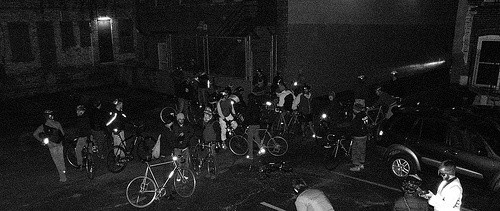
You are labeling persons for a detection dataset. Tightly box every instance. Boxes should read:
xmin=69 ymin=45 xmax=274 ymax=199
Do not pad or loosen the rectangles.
xmin=390 ymin=174 xmax=429 ymax=211
xmin=291 ymin=178 xmax=335 ymax=211
xmin=33 ymin=66 xmax=411 ymax=182
xmin=419 ymin=160 xmax=463 ymax=211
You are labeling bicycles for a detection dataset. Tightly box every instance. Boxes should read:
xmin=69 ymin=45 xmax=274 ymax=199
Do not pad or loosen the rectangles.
xmin=106 ymin=129 xmax=158 ymax=174
xmin=126 ymin=155 xmax=196 ymax=209
xmin=66 ymin=130 xmax=96 ymax=180
xmin=325 ymin=135 xmax=354 ymax=171
xmin=272 ymin=110 xmax=301 ymax=138
xmin=159 ymin=99 xmax=202 ymax=126
xmin=228 ymin=126 xmax=289 ymax=157
xmin=189 ymin=136 xmax=217 ymax=179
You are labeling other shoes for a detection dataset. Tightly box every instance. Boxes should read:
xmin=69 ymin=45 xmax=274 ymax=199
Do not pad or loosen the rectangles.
xmin=258 ymin=147 xmax=265 ymax=154
xmin=222 ymin=144 xmax=227 ymax=150
xmin=350 ymin=166 xmax=360 ymax=171
xmin=359 ymin=164 xmax=364 ymax=169
xmin=246 ymin=156 xmax=253 ymax=160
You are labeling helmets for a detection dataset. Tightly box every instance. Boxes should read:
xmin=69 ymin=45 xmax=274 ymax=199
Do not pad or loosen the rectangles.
xmin=439 ymin=161 xmax=455 ymax=175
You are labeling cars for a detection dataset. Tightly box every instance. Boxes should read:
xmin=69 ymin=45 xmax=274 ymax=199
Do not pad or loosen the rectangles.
xmin=370 ymin=114 xmax=500 ymax=198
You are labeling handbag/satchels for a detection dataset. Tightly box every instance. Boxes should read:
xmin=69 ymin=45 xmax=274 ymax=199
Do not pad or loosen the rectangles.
xmin=175 ymin=148 xmax=190 ymax=169
xmin=152 ymin=134 xmax=162 ymax=159
xmin=43 ymin=123 xmax=63 ymax=144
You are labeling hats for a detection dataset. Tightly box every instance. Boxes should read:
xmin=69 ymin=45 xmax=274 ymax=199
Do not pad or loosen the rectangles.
xmin=328 ymin=91 xmax=335 ymax=97
xmin=177 ymin=113 xmax=185 ymax=121
xmin=43 ymin=110 xmax=54 ymax=114
xmin=277 ymin=79 xmax=284 ymax=84
xmin=204 ymin=107 xmax=213 ymax=115
xmin=353 ymin=104 xmax=363 ymax=111
xmin=374 ymin=87 xmax=384 ymax=91
xmin=76 ymin=105 xmax=86 ymax=110
xmin=304 ymin=87 xmax=309 ymax=90
